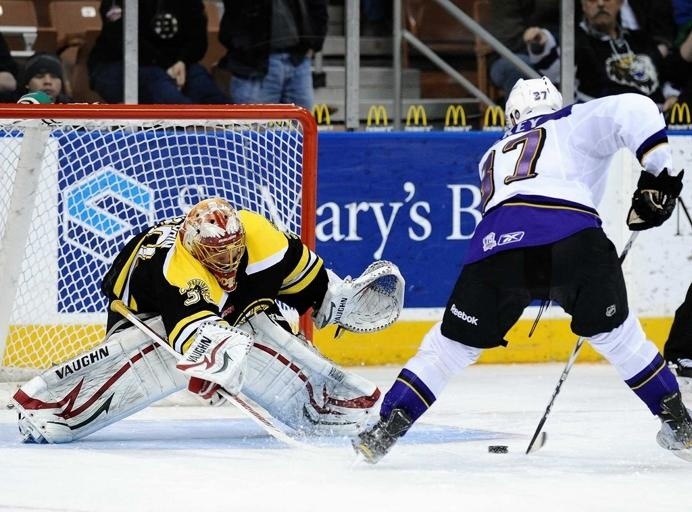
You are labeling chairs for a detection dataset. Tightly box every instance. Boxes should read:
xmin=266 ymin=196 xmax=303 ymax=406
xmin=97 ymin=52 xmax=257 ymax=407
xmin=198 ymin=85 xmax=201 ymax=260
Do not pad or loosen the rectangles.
xmin=0 ymin=0 xmax=241 ymax=113
xmin=392 ymin=1 xmax=540 ymax=131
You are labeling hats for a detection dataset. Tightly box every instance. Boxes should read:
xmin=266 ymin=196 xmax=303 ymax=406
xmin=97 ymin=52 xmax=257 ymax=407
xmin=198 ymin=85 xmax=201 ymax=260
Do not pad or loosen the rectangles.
xmin=21 ymin=51 xmax=64 ymax=86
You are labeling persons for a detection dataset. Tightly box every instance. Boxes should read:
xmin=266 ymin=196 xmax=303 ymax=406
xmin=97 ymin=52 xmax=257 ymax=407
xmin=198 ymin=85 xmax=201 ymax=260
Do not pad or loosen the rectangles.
xmin=665 ymin=280 xmax=690 ymax=378
xmin=342 ymin=1 xmax=413 ymax=69
xmin=215 ymin=1 xmax=329 ymax=112
xmin=349 ymin=74 xmax=691 ymax=462
xmin=1 ymin=32 xmax=74 ymax=104
xmin=483 ymin=0 xmax=692 ymax=125
xmin=11 ymin=199 xmax=405 ymax=444
xmin=88 ymin=1 xmax=235 ymax=104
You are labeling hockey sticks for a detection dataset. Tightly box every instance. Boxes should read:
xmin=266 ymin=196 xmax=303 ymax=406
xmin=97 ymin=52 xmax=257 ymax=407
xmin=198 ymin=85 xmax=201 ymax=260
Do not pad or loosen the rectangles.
xmin=111 ymin=301 xmax=360 ymax=449
xmin=527 ymin=227 xmax=639 ymax=454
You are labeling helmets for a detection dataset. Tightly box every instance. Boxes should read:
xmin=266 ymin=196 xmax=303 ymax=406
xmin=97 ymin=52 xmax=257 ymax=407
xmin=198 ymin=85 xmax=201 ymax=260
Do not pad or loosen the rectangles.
xmin=177 ymin=195 xmax=250 ymax=294
xmin=502 ymin=75 xmax=567 ymax=131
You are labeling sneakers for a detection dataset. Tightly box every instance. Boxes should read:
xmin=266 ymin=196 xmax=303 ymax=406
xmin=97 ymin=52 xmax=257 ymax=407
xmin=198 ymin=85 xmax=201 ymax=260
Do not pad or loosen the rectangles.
xmin=655 ymin=390 xmax=692 ymax=449
xmin=671 ymin=358 xmax=692 ymax=378
xmin=350 ymin=407 xmax=414 ymax=465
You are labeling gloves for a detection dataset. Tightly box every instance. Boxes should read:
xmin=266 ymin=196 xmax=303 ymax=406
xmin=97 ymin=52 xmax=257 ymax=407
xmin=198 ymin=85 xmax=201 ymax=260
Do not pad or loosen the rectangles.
xmin=626 ymin=168 xmax=686 ymax=232
